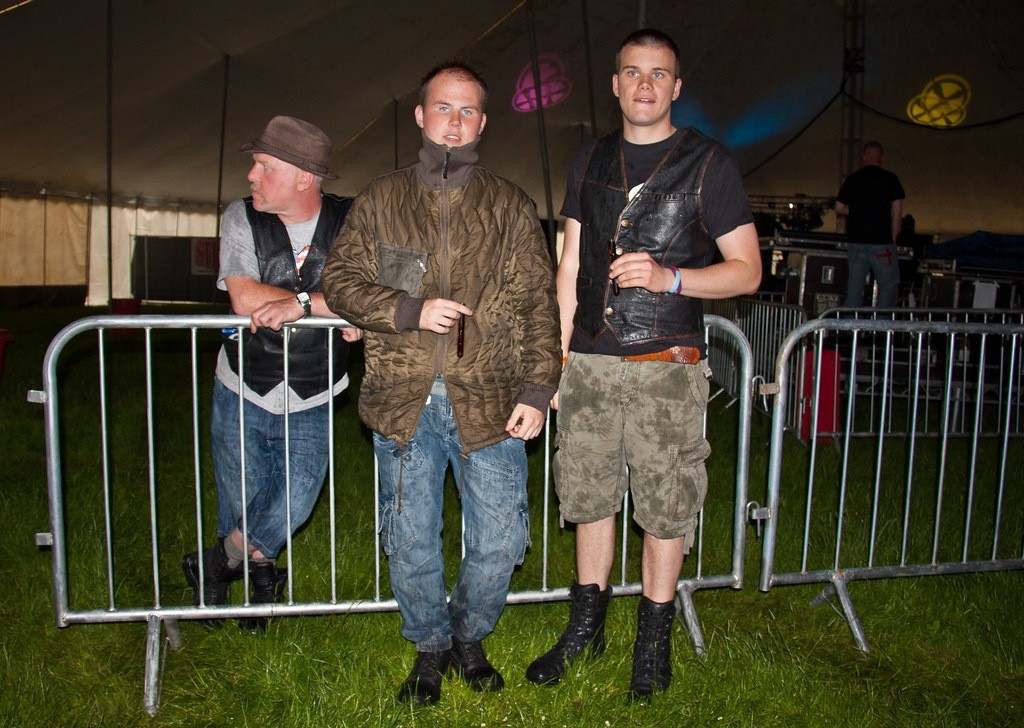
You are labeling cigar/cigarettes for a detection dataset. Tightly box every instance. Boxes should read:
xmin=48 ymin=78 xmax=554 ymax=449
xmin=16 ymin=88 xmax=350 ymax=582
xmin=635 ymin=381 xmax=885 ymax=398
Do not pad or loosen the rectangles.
xmin=457 ymin=303 xmax=466 ymax=358
xmin=610 ymin=239 xmax=620 ymax=296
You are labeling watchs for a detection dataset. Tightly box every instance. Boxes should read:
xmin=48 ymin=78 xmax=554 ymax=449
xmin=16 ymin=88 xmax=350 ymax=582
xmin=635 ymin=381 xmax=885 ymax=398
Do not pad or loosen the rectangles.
xmin=296 ymin=291 xmax=312 ymax=316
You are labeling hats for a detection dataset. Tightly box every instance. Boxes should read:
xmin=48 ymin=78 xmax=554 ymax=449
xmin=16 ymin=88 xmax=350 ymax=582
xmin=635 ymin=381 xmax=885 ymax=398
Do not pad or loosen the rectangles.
xmin=238 ymin=115 xmax=339 ymax=180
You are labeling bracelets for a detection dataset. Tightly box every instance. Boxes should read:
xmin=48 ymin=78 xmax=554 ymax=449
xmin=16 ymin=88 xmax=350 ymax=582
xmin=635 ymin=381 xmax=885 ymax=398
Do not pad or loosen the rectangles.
xmin=667 ymin=267 xmax=681 ymax=296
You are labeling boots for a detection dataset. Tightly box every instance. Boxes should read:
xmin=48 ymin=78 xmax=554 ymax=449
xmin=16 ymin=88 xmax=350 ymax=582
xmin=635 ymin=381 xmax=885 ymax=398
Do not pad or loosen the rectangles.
xmin=526 ymin=580 xmax=613 ymax=687
xmin=626 ymin=597 xmax=677 ymax=705
xmin=182 ymin=536 xmax=244 ymax=631
xmin=238 ymin=561 xmax=288 ymax=633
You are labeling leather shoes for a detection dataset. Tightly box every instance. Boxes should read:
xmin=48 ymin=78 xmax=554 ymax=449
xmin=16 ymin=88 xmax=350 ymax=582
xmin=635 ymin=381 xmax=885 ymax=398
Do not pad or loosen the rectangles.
xmin=451 ymin=635 xmax=505 ymax=693
xmin=398 ymin=651 xmax=450 ymax=707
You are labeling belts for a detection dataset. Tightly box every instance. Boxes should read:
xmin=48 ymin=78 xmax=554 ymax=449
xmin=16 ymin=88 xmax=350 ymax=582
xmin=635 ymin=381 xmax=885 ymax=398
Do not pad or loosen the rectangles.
xmin=623 ymin=346 xmax=700 ymax=366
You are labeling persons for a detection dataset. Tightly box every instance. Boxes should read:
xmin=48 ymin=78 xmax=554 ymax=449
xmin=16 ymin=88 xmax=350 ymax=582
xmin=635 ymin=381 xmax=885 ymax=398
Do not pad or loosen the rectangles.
xmin=321 ymin=65 xmax=563 ymax=703
xmin=525 ymin=31 xmax=762 ymax=704
xmin=183 ymin=113 xmax=363 ymax=634
xmin=834 ymin=141 xmax=906 ymax=320
